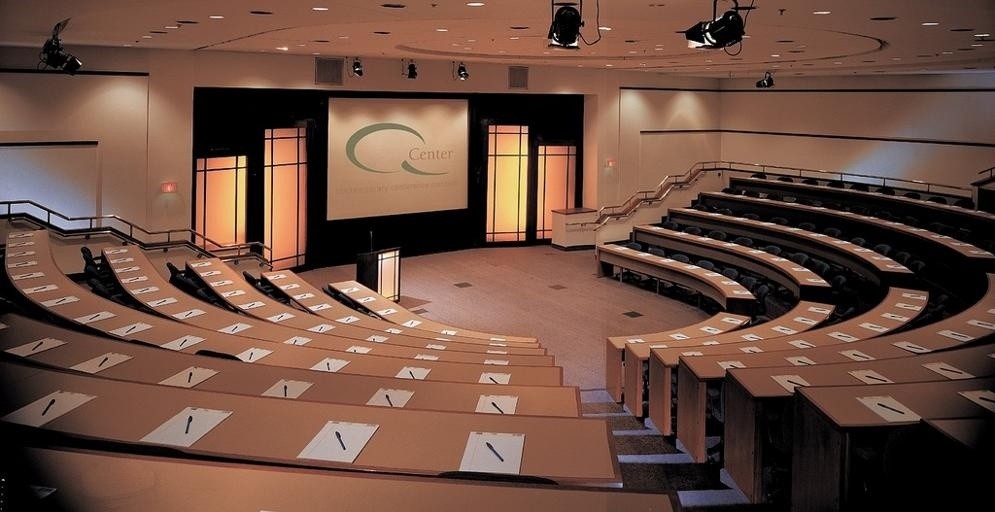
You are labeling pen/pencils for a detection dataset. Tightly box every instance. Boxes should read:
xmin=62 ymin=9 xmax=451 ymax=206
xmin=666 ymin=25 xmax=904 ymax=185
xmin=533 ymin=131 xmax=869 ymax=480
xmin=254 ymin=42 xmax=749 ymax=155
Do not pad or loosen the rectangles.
xmin=910 ymin=295 xmax=921 ymax=297
xmin=229 ymin=292 xmax=236 ymax=295
xmin=489 ymin=377 xmax=498 ymax=384
xmin=977 ymin=323 xmax=992 ymax=326
xmin=951 ymin=333 xmax=968 ymax=338
xmin=801 ymin=319 xmax=811 ymax=321
xmin=877 ymin=402 xmax=904 ymax=414
xmin=729 ymin=364 xmax=738 ymax=368
xmin=185 ymin=415 xmax=192 ymax=433
xmin=870 ymin=326 xmax=881 ymax=328
xmin=409 ymin=371 xmax=415 ymax=379
xmin=787 ymin=380 xmax=805 ymax=387
xmin=42 ymin=399 xmax=55 ymax=415
xmin=32 ymin=341 xmax=43 ymax=351
xmin=34 ymin=287 xmax=46 ymax=291
xmin=16 ymin=261 xmax=28 ymax=266
xmin=491 ymin=402 xmax=503 ymax=414
xmin=21 ymin=274 xmax=33 ymax=277
xmin=56 ymin=298 xmax=65 ymax=303
xmin=199 ymin=263 xmax=205 ymax=265
xmin=319 ymin=326 xmax=323 ymax=331
xmin=140 ymin=288 xmax=149 ymax=292
xmin=207 ymin=272 xmax=213 ymax=275
xmin=797 ymin=360 xmax=811 ymax=366
xmin=16 ymin=243 xmax=25 ymax=245
xmin=248 ymin=304 xmax=254 ymax=307
xmin=15 ymin=252 xmax=25 ymax=256
xmin=283 ymin=384 xmax=287 ymax=396
xmin=278 ymin=315 xmax=283 ymax=320
xmin=119 ymin=258 xmax=126 ymax=261
xmin=232 ymin=326 xmax=238 ymax=331
xmin=385 ymin=394 xmax=393 ymax=407
xmin=800 ymin=343 xmax=813 ymax=348
xmin=815 ymin=309 xmax=826 ymax=311
xmin=939 ymin=368 xmax=962 ymax=374
xmin=891 ymin=315 xmax=903 ymax=318
xmin=185 ymin=312 xmax=192 ymax=317
xmin=979 ymin=397 xmax=995 ymax=403
xmin=124 ymin=268 xmax=131 ymax=270
xmin=126 ymin=326 xmax=136 ymax=333
xmin=749 ymin=350 xmax=756 ymax=352
xmin=293 ymin=340 xmax=296 ymax=345
xmin=865 ymin=376 xmax=887 ymax=382
xmin=157 ymin=300 xmax=166 ymax=304
xmin=750 ymin=336 xmax=762 ymax=340
xmin=112 ymin=250 xmax=119 ymax=253
xmin=188 ymin=372 xmax=192 ymax=383
xmin=906 ymin=346 xmax=923 ymax=351
xmin=837 ymin=334 xmax=851 ymax=338
xmin=335 ymin=431 xmax=346 ymax=451
xmin=852 ymin=354 xmax=869 ymax=359
xmin=904 ymin=305 xmax=915 ymax=307
xmin=485 ymin=442 xmax=504 ymax=462
xmin=327 ymin=362 xmax=330 ymax=370
xmin=129 ymin=278 xmax=139 ymax=281
xmin=89 ymin=314 xmax=100 ymax=320
xmin=780 ymin=328 xmax=791 ymax=331
xmin=218 ymin=281 xmax=224 ymax=285
xmin=17 ymin=234 xmax=24 ymax=236
xmin=180 ymin=339 xmax=186 ymax=346
xmin=249 ymin=352 xmax=253 ymax=360
xmin=98 ymin=357 xmax=108 ymax=367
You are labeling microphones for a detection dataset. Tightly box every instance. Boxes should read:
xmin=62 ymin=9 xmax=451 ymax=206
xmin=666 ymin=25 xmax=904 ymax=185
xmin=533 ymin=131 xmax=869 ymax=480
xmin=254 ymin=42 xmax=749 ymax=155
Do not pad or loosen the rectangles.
xmin=370 ymin=231 xmax=372 ymax=253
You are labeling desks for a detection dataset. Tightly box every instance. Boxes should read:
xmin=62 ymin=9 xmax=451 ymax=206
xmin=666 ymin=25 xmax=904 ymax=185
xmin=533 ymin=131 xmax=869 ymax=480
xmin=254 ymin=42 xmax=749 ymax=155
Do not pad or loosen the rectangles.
xmin=550 ymin=206 xmax=599 ymax=252
xmin=0 ymin=171 xmax=995 ymax=510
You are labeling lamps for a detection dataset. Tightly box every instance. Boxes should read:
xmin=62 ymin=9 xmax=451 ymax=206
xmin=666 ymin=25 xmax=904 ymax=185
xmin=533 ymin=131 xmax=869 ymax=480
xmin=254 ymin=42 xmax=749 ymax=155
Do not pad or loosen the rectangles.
xmin=344 ymin=55 xmax=363 ymax=78
xmin=35 ymin=23 xmax=83 ymax=76
xmin=161 ymin=182 xmax=179 ymax=193
xmin=451 ymin=59 xmax=469 ymax=82
xmin=676 ymin=1 xmax=755 ymax=55
xmin=546 ymin=0 xmax=602 ymax=52
xmin=400 ymin=59 xmax=419 ymax=79
xmin=755 ymin=71 xmax=774 ymax=88
